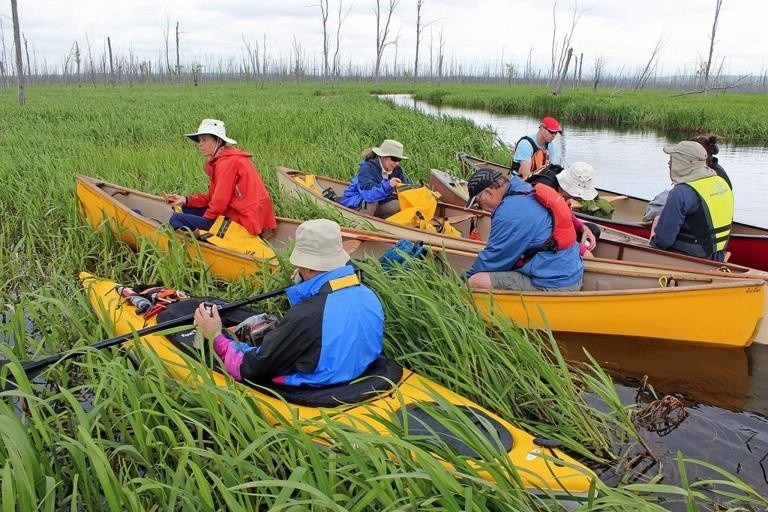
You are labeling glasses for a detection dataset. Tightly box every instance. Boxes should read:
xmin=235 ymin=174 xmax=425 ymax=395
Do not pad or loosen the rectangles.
xmin=476 ymin=193 xmax=483 ymax=209
xmin=389 ymin=156 xmax=402 ymax=162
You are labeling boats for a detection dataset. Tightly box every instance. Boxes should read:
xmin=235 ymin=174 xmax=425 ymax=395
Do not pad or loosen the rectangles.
xmin=275 ymin=166 xmax=768 ymax=347
xmin=427 ymin=167 xmax=653 ymax=249
xmin=79 ymin=271 xmax=598 ymax=512
xmin=456 ymin=150 xmax=768 ymax=273
xmin=743 ymin=342 xmax=768 ymax=422
xmin=73 ymin=172 xmax=766 ymax=349
xmin=485 ymin=330 xmax=749 ymax=413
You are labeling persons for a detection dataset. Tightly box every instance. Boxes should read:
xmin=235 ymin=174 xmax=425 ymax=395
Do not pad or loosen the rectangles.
xmin=193 ymin=218 xmax=384 ymax=389
xmin=688 ymin=134 xmax=733 ymax=191
xmin=648 ymin=141 xmax=733 ymax=262
xmin=522 ymin=163 xmax=601 ymax=260
xmin=507 ymin=117 xmax=562 ymax=182
xmin=452 ymin=168 xmax=584 ymax=294
xmin=339 ymin=139 xmax=409 ymax=219
xmin=165 ymin=119 xmax=276 ymax=244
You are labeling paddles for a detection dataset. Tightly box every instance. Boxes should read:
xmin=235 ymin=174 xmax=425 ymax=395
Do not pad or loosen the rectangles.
xmin=0 ymin=260 xmax=373 ymax=398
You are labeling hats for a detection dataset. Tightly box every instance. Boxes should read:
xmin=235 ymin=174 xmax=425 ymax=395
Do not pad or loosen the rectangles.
xmin=372 ymin=140 xmax=408 ymax=160
xmin=663 ymin=142 xmax=708 ymax=160
xmin=290 ymin=219 xmax=350 ymax=272
xmin=464 ymin=167 xmax=504 ymax=210
xmin=556 ymin=162 xmax=599 ymax=201
xmin=185 ymin=118 xmax=238 ymax=144
xmin=541 ymin=117 xmax=563 ymax=134
